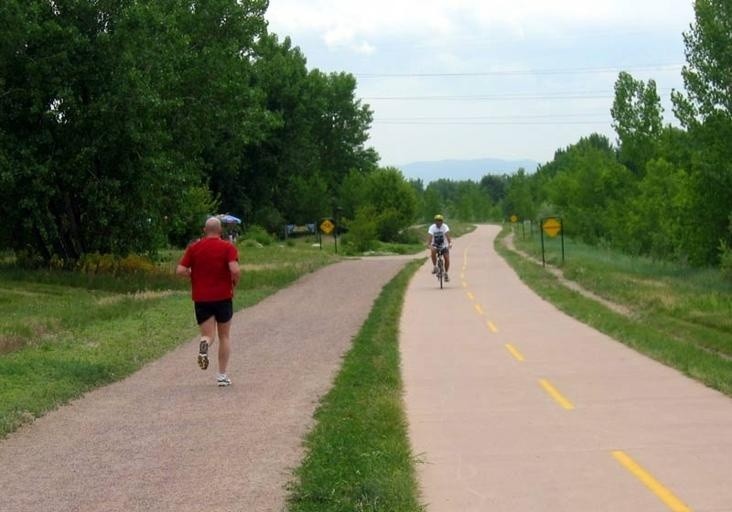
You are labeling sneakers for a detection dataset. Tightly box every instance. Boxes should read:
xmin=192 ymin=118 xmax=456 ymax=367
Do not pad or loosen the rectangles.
xmin=431 ymin=266 xmax=438 ymax=274
xmin=197 ymin=337 xmax=211 ymax=370
xmin=444 ymin=273 xmax=450 ymax=282
xmin=216 ymin=376 xmax=233 ymax=387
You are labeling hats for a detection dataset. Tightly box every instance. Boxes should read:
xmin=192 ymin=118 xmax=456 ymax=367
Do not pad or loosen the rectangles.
xmin=434 ymin=214 xmax=444 ymax=221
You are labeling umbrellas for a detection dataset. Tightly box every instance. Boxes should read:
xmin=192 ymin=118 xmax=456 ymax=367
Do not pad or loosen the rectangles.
xmin=208 ymin=212 xmax=243 ymax=239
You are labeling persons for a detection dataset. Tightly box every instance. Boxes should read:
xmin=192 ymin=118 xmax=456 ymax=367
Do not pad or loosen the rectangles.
xmin=426 ymin=214 xmax=453 ymax=283
xmin=176 ymin=216 xmax=241 ymax=387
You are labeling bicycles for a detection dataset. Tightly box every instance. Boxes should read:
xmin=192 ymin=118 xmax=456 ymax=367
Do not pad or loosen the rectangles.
xmin=426 ymin=245 xmax=453 ymax=288
